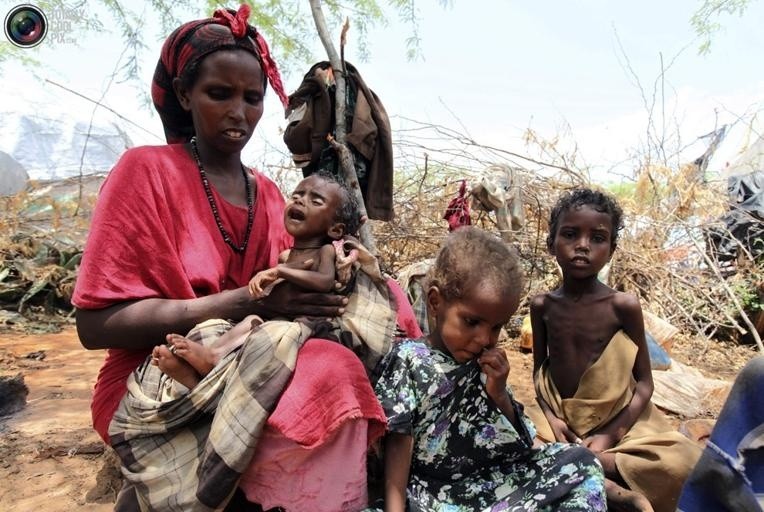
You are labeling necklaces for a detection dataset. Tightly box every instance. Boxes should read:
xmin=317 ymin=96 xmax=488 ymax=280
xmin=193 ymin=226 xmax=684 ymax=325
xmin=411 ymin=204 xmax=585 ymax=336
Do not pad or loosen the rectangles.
xmin=287 ymin=243 xmax=320 ymax=252
xmin=190 ymin=141 xmax=254 ymax=259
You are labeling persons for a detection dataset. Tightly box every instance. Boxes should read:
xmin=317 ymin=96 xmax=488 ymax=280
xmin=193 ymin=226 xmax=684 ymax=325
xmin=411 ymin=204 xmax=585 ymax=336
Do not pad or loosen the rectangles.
xmin=526 ymin=188 xmax=708 ymax=512
xmin=150 ymin=164 xmax=367 ymax=393
xmin=71 ymin=2 xmax=389 ymax=511
xmin=364 ymin=221 xmax=608 ymax=512
